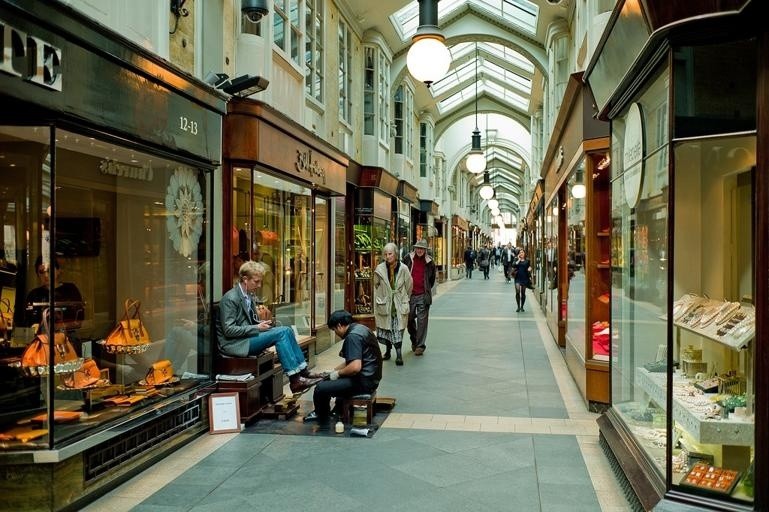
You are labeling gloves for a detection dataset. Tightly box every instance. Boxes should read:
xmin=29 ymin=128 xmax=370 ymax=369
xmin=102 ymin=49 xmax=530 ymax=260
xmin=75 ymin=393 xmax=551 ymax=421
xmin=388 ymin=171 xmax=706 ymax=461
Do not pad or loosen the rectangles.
xmin=320 ymin=369 xmax=335 ymax=378
xmin=329 ymin=370 xmax=339 ymax=382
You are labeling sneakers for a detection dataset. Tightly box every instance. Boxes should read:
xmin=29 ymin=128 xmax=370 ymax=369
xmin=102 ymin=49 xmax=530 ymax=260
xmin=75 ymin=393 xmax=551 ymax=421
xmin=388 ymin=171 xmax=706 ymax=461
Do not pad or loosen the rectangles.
xmin=414 ymin=347 xmax=425 ymax=356
xmin=412 ymin=343 xmax=417 ymax=352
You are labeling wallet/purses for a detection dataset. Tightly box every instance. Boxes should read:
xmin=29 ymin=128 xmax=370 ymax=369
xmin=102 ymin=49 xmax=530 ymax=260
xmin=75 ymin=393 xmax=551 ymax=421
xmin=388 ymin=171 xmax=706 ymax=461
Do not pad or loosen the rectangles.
xmin=104 ymin=393 xmax=145 ymax=407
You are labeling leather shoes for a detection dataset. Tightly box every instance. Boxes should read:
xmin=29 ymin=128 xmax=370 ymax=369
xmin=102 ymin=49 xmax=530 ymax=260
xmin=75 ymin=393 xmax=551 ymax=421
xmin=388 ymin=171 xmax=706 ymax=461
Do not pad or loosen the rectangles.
xmin=303 ymin=410 xmax=319 ymax=422
xmin=289 ymin=375 xmax=322 ymax=394
xmin=300 ymin=370 xmax=325 ymax=379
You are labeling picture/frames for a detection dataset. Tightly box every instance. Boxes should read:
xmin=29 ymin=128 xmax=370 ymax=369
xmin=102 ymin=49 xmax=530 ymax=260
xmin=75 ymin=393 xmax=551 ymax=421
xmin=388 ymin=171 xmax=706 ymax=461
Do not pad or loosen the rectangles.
xmin=209 ymin=391 xmax=241 ymax=435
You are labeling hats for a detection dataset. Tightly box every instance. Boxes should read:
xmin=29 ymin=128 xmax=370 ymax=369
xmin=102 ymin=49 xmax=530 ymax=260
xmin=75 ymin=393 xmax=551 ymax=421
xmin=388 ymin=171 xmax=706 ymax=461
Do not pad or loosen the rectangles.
xmin=412 ymin=238 xmax=431 ymax=250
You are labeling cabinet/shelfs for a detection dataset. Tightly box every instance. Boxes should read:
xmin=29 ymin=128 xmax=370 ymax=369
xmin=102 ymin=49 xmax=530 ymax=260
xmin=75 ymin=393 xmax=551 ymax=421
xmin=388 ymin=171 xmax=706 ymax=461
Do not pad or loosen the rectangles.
xmin=634 ymin=292 xmax=755 ymax=471
xmin=355 ymin=247 xmax=382 ymax=314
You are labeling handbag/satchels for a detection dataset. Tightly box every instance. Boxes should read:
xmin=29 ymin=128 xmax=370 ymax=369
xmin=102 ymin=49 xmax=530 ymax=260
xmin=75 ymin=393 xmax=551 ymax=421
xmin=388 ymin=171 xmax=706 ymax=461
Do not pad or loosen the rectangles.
xmin=106 ymin=298 xmax=151 ymax=348
xmin=21 ymin=308 xmax=78 ymax=367
xmin=145 ymin=360 xmax=173 ymax=385
xmin=61 ymin=360 xmax=102 ymax=389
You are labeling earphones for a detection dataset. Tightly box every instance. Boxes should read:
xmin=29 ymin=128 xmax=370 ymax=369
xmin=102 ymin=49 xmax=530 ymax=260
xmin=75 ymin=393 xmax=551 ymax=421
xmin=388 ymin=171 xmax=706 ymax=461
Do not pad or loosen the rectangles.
xmin=244 ymin=279 xmax=247 ymax=284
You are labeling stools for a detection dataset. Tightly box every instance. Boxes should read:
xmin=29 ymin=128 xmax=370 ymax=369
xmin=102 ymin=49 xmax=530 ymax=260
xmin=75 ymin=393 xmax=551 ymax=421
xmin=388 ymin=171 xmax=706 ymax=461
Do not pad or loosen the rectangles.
xmin=343 ymin=391 xmax=376 ymax=426
xmin=194 ymin=351 xmax=300 ymax=427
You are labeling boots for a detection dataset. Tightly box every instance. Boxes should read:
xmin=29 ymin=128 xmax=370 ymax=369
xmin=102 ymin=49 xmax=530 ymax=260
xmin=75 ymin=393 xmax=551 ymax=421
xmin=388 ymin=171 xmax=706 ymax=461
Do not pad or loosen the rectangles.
xmin=382 ymin=349 xmax=392 ymax=360
xmin=395 ymin=348 xmax=403 ymax=366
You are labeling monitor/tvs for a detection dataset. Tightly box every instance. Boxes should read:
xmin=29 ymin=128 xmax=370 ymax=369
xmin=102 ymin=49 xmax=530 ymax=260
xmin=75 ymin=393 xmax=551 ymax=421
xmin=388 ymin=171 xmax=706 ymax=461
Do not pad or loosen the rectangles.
xmin=44 ymin=216 xmax=100 ymax=257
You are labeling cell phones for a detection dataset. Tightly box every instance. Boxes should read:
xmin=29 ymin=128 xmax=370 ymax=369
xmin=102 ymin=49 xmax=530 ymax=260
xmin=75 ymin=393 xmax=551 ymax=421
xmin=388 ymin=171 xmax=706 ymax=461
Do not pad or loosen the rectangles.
xmin=268 ymin=321 xmax=274 ymax=325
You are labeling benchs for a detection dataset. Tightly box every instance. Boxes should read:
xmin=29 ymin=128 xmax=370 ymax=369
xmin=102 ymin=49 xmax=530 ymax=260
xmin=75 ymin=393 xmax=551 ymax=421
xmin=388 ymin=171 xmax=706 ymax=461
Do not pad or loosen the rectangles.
xmin=271 ymin=334 xmax=317 ymax=385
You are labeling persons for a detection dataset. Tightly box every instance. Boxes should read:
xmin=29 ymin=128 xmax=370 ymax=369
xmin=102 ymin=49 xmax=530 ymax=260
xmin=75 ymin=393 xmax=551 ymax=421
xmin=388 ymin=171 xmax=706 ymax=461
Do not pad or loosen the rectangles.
xmin=25 ymin=251 xmax=84 ymax=327
xmin=402 ymin=238 xmax=436 ymax=355
xmin=156 ymin=257 xmax=210 ymax=373
xmin=511 ymin=249 xmax=532 ymax=312
xmin=215 ymin=260 xmax=333 ymax=393
xmin=463 ymin=241 xmax=522 ymax=284
xmin=371 ymin=243 xmax=413 ymax=365
xmin=302 ymin=309 xmax=383 ymax=431
xmin=57 ymin=252 xmax=66 ymax=280
xmin=546 ymin=243 xmax=573 ymax=299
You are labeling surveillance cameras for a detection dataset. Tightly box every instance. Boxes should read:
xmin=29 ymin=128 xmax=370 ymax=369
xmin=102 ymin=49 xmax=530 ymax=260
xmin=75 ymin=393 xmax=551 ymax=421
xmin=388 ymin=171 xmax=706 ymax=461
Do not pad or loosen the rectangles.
xmin=241 ymin=0 xmax=270 ymax=24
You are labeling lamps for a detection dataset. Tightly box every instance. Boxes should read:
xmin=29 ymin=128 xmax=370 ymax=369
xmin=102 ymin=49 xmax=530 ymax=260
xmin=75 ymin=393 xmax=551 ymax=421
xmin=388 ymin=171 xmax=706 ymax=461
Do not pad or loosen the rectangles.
xmin=405 ymin=0 xmax=452 ymax=88
xmin=479 ymin=166 xmax=494 ymax=200
xmin=487 ymin=188 xmax=498 ymax=209
xmin=571 ymin=169 xmax=586 ymax=199
xmin=466 ymin=42 xmax=487 ymax=179
